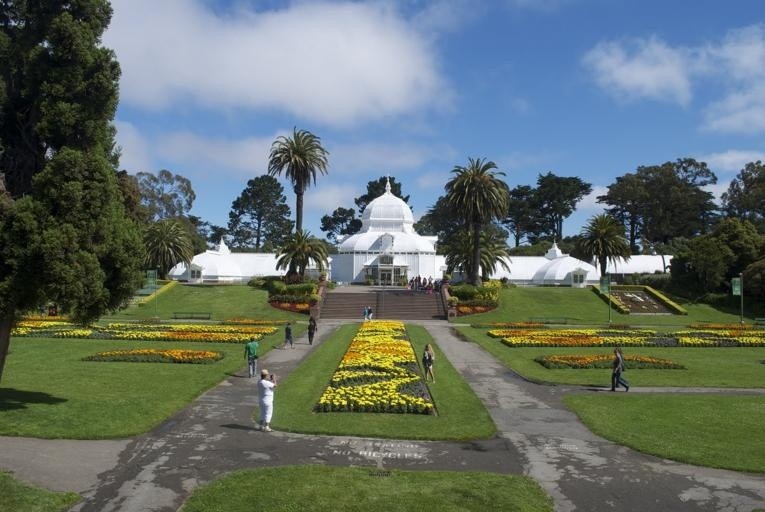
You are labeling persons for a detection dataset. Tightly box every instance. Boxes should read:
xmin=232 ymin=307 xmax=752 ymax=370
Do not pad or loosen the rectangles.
xmin=308 ymin=316 xmax=317 ymax=345
xmin=284 ymin=323 xmax=294 ymax=349
xmin=244 ymin=336 xmax=259 ymax=378
xmin=610 ymin=348 xmax=629 ymax=392
xmin=258 ymin=370 xmax=277 ymax=432
xmin=364 ymin=306 xmax=372 ymax=320
xmin=409 ymin=275 xmax=439 ymax=296
xmin=422 ymin=344 xmax=435 ymax=384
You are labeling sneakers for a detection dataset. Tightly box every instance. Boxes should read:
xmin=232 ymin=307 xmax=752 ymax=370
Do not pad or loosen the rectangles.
xmin=425 ymin=377 xmax=435 ymax=384
xmin=611 ymin=381 xmax=630 ymax=392
xmin=258 ymin=424 xmax=274 ymax=432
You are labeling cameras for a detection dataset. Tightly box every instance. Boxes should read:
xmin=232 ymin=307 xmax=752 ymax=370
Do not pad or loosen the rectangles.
xmin=270 ymin=375 xmax=273 ymax=380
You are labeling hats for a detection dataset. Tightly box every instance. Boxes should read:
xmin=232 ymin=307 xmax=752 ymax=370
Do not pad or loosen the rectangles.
xmin=261 ymin=369 xmax=270 ymax=376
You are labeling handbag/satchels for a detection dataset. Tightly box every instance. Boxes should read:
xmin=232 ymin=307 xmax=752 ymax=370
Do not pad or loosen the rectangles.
xmin=422 ymin=355 xmax=432 ymax=368
